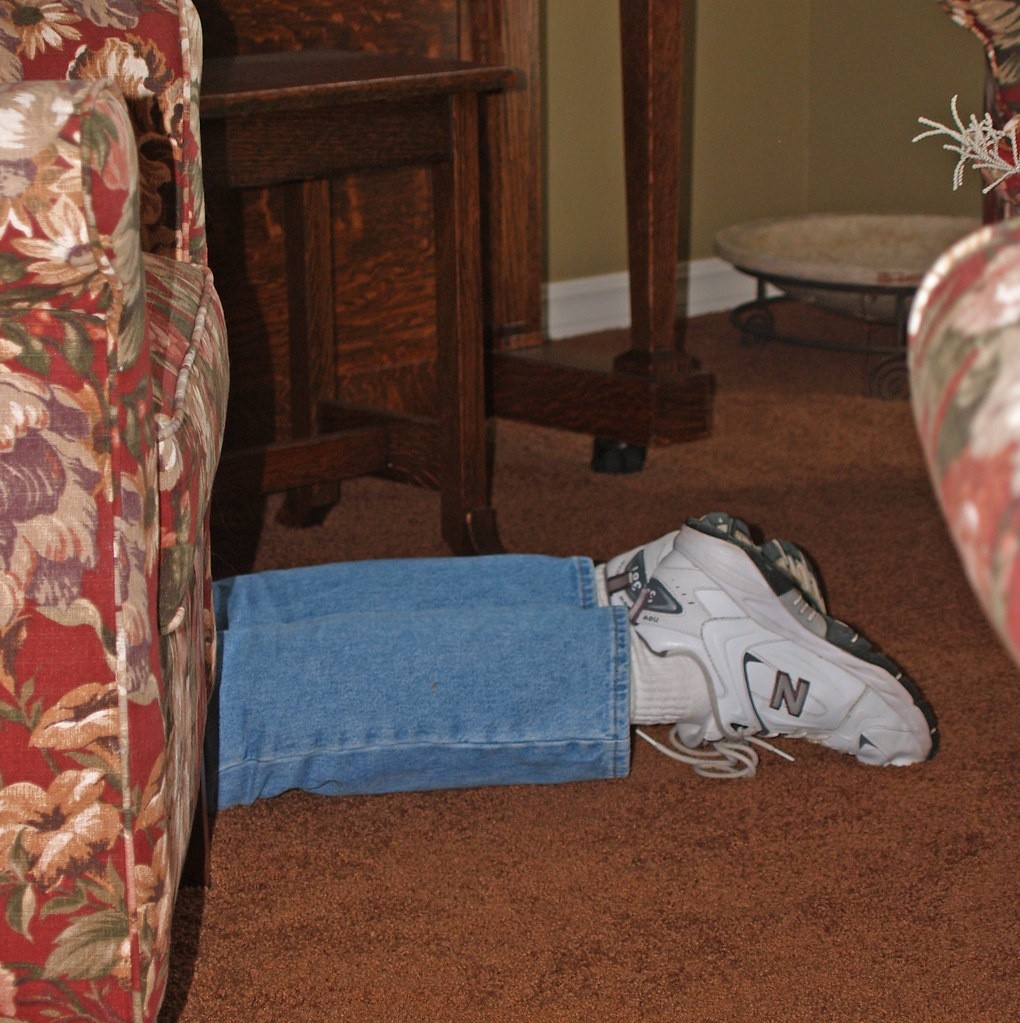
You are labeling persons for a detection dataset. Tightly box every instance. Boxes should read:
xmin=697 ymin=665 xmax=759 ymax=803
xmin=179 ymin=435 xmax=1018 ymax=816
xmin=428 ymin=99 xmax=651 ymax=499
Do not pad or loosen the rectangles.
xmin=204 ymin=511 xmax=941 ymax=816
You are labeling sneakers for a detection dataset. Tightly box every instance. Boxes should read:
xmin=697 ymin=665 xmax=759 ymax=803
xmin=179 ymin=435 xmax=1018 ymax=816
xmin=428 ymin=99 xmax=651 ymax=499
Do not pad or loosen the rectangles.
xmin=603 ymin=511 xmax=826 ymax=663
xmin=628 ymin=516 xmax=939 ymax=779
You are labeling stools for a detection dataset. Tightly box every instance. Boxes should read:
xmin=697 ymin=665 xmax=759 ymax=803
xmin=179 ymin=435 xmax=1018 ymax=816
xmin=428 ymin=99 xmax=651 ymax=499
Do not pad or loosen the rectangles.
xmin=198 ymin=51 xmax=524 ymax=564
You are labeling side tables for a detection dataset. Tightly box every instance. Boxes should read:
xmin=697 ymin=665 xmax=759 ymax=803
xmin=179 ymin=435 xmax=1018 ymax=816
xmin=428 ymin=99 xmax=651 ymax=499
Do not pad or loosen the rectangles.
xmin=190 ymin=0 xmax=722 ymax=481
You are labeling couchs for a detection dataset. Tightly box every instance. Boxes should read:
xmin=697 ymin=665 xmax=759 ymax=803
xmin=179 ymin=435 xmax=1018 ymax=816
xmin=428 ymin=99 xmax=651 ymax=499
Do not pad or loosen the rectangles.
xmin=893 ymin=0 xmax=1020 ymax=673
xmin=0 ymin=0 xmax=238 ymax=1023
